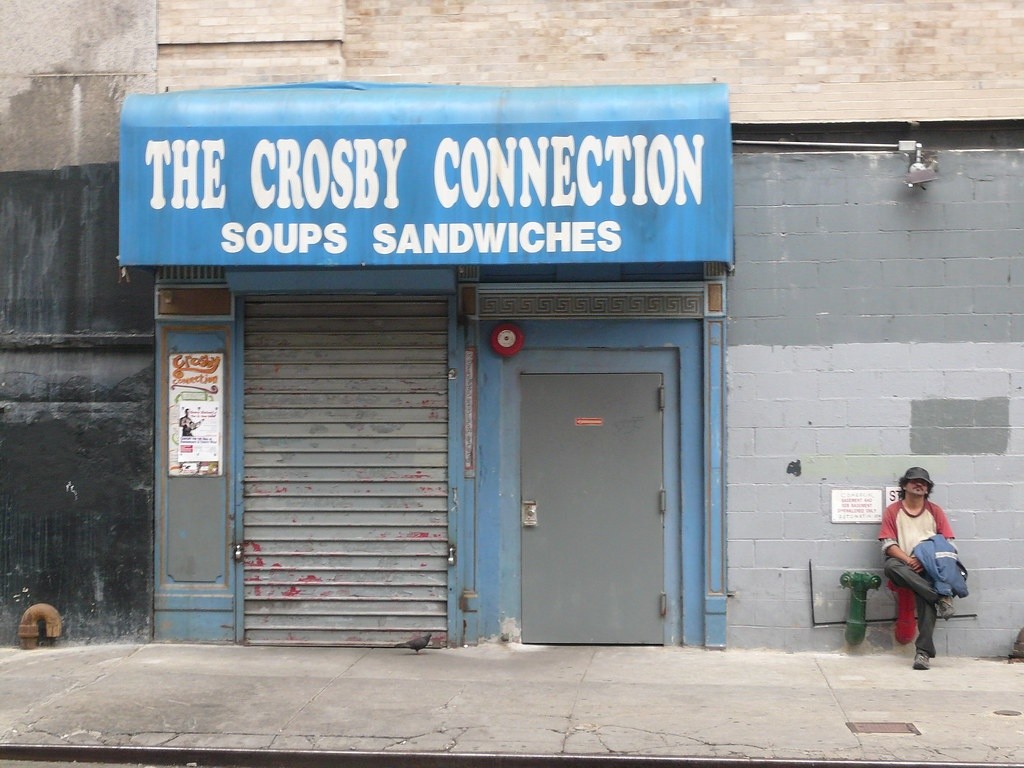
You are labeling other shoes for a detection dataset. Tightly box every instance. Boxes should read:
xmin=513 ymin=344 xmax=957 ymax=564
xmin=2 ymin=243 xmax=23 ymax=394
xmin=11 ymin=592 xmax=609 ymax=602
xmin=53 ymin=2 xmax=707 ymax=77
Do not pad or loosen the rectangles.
xmin=913 ymin=654 xmax=930 ymax=669
xmin=937 ymin=595 xmax=956 ymax=620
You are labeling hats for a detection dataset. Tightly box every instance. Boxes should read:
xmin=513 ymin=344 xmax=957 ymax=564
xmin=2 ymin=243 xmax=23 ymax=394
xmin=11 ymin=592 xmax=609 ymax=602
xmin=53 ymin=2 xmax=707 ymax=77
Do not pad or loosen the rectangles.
xmin=905 ymin=467 xmax=931 ymax=484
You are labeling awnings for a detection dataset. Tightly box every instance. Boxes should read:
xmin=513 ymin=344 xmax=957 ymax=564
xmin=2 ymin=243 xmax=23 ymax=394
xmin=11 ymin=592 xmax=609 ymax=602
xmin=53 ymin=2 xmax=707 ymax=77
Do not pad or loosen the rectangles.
xmin=118 ymin=78 xmax=740 ymax=281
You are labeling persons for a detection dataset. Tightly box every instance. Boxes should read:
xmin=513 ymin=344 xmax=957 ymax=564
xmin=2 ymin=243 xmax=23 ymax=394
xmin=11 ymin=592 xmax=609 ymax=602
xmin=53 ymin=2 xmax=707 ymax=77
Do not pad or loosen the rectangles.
xmin=877 ymin=467 xmax=969 ymax=672
xmin=179 ymin=409 xmax=196 ymax=437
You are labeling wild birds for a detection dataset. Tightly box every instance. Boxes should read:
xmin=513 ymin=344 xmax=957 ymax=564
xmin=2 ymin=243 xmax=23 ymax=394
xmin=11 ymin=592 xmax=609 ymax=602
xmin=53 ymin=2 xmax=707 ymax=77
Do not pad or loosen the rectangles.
xmin=395 ymin=633 xmax=433 ymax=655
